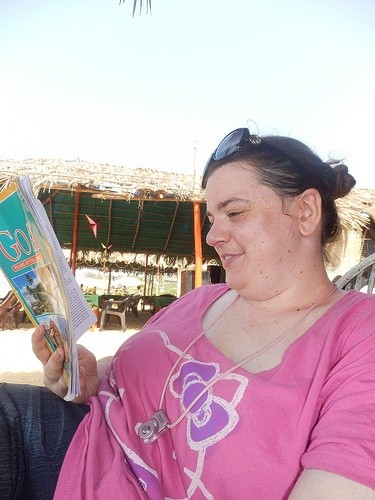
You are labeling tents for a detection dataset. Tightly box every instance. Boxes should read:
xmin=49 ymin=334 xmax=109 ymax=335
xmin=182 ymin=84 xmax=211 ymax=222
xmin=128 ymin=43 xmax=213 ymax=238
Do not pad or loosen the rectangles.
xmin=0 ymin=158 xmax=375 ymax=290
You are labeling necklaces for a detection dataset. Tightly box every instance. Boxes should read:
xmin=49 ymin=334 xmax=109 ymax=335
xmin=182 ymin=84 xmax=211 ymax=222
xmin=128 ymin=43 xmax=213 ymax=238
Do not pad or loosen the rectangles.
xmin=137 ymin=283 xmax=337 ymax=446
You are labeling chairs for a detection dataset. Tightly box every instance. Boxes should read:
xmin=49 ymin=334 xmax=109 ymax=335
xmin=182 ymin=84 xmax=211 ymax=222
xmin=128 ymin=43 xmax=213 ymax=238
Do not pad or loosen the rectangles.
xmin=0 ymin=290 xmax=142 ymax=331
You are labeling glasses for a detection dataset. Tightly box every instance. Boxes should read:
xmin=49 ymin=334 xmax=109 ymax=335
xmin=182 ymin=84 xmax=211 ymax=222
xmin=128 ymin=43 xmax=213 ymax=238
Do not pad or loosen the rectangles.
xmin=201 ymin=128 xmax=314 ymax=188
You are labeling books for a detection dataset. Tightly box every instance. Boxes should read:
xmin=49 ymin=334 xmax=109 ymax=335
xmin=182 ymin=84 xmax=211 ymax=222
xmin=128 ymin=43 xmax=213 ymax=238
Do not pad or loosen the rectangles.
xmin=0 ymin=176 xmax=97 ymax=401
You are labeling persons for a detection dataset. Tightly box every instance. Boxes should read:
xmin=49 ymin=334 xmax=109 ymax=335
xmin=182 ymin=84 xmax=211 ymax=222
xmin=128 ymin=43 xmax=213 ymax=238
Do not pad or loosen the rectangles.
xmin=0 ymin=137 xmax=375 ymax=500
